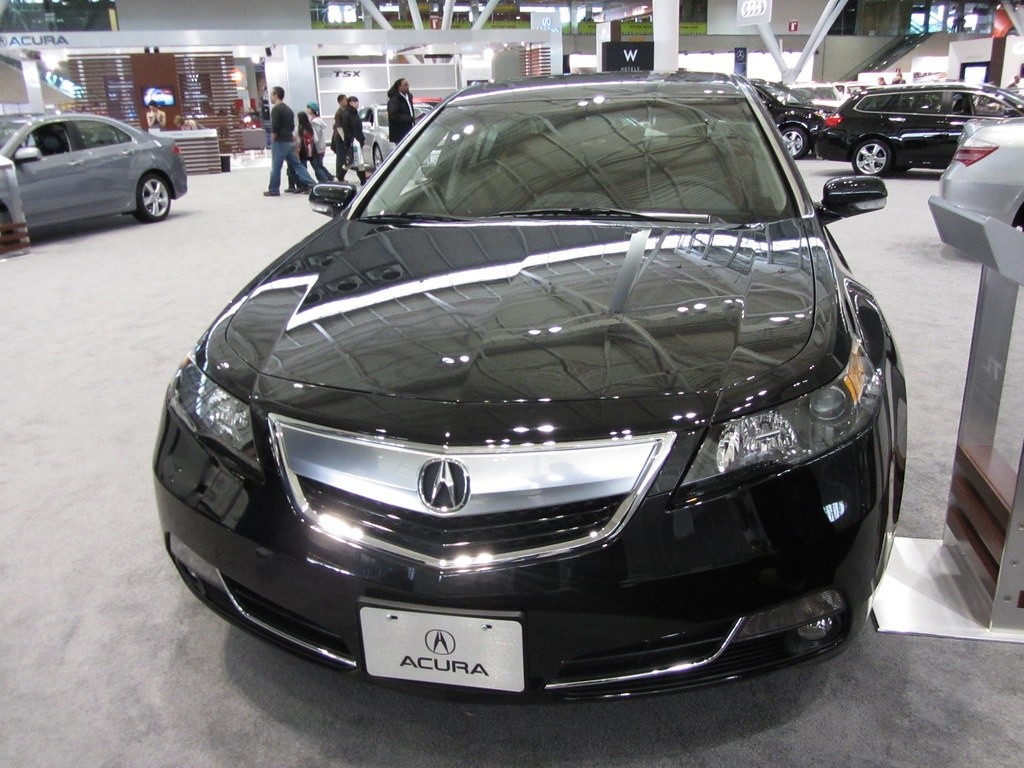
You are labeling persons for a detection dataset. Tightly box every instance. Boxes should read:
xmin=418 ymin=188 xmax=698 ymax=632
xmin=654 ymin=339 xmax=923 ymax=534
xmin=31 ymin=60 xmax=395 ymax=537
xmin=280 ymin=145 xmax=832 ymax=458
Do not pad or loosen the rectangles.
xmin=301 ymin=102 xmax=333 ymax=182
xmin=264 ymin=86 xmax=317 ymax=196
xmin=336 ymin=96 xmax=367 ymax=186
xmin=333 ymin=95 xmax=348 ymax=184
xmin=1008 ymin=74 xmax=1021 ymax=88
xmin=146 ymin=100 xmax=167 ymax=131
xmin=174 ymin=115 xmax=207 ymax=130
xmin=877 ymin=72 xmax=906 ymax=86
xmin=285 ymin=112 xmax=331 ymax=192
xmin=387 ymin=78 xmax=417 ymax=147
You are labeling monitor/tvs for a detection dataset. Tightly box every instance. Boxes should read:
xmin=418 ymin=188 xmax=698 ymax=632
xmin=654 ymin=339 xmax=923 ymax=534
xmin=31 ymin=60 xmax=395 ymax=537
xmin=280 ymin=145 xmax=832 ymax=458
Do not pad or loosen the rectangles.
xmin=141 ymin=85 xmax=176 ymax=108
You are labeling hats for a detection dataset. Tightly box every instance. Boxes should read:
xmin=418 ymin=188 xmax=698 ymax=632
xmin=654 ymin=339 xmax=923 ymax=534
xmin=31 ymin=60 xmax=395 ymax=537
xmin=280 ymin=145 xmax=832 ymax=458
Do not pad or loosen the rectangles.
xmin=307 ymin=101 xmax=318 ymax=112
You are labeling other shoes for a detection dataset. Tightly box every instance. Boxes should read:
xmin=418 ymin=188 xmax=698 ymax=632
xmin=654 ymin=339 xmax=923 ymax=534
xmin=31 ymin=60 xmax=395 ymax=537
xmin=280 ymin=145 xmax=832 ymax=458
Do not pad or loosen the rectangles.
xmin=294 ymin=188 xmax=309 ymax=193
xmin=263 ymin=191 xmax=280 ymax=196
xmin=285 ymin=188 xmax=295 ymax=192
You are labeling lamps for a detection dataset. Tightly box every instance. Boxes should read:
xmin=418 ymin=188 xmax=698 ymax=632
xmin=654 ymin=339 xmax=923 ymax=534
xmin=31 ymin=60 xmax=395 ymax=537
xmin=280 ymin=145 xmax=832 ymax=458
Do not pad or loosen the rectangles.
xmin=326 ymin=2 xmax=357 ymax=23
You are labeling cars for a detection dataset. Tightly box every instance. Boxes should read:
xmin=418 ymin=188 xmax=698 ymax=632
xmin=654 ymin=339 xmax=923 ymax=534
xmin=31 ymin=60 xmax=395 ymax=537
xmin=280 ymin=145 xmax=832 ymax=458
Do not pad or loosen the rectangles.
xmin=939 ymin=117 xmax=1024 ymax=232
xmin=152 ymin=72 xmax=910 ymax=706
xmin=814 ymin=81 xmax=1024 ymax=179
xmin=748 ymin=78 xmax=878 ymax=161
xmin=0 ymin=113 xmax=189 ymax=230
xmin=357 ymin=103 xmax=448 ymax=178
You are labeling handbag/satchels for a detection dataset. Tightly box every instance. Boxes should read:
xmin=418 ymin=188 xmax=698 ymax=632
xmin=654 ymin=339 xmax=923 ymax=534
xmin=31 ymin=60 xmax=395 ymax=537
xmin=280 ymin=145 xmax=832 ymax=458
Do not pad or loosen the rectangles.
xmin=342 ymin=137 xmax=365 ymax=171
